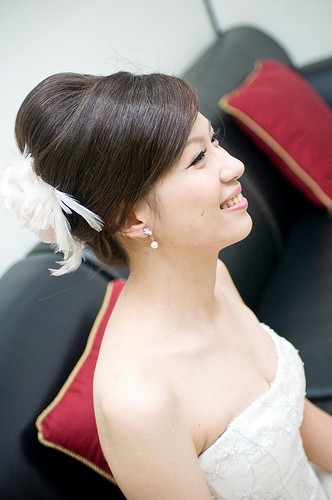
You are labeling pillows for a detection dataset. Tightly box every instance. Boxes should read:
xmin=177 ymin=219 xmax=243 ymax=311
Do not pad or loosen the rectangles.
xmin=218 ymin=56 xmax=332 ymax=211
xmin=36 ymin=278 xmax=116 ymax=485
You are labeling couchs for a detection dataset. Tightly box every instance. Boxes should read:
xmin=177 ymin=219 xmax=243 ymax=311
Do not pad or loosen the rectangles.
xmin=0 ymin=25 xmax=331 ymax=500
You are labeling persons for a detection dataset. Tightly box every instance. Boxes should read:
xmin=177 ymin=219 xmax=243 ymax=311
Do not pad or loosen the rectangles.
xmin=15 ymin=48 xmax=332 ymax=500
